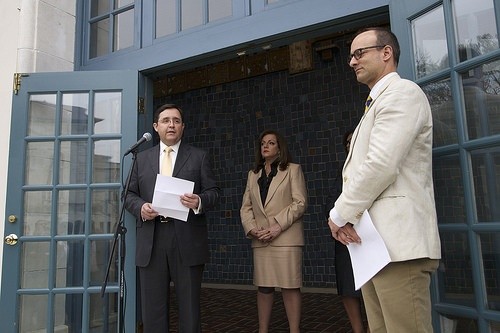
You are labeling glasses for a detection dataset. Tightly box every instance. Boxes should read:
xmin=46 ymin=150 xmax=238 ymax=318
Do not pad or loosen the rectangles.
xmin=346 ymin=45 xmax=386 ymax=65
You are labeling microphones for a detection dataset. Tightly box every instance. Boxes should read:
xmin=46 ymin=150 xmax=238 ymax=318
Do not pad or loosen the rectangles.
xmin=123 ymin=132 xmax=152 ymax=156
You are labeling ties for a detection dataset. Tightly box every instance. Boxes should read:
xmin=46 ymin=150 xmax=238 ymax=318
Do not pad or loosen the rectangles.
xmin=364 ymin=95 xmax=372 ymax=113
xmin=161 ymin=147 xmax=173 ymax=177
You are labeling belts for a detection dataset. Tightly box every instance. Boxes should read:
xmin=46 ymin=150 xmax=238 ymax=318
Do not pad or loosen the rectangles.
xmin=155 ymin=215 xmax=174 ymax=222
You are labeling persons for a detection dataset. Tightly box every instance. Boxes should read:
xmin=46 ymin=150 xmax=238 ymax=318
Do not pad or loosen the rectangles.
xmin=121 ymin=104 xmax=221 ymax=333
xmin=328 ymin=27 xmax=441 ymax=333
xmin=240 ymin=130 xmax=307 ymax=333
xmin=324 ymin=126 xmax=371 ymax=333
